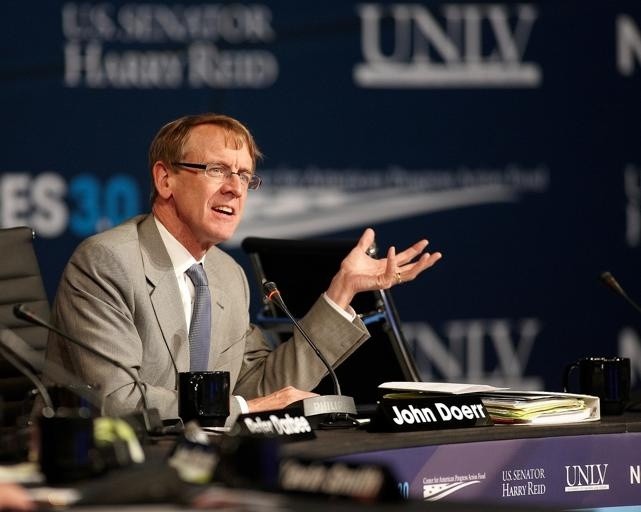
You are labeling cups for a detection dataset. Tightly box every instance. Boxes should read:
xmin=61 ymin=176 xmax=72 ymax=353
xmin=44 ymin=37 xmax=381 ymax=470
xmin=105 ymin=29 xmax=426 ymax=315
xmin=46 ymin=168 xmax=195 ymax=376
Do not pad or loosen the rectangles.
xmin=176 ymin=370 xmax=231 ymax=426
xmin=562 ymin=356 xmax=631 ymax=416
xmin=35 ymin=408 xmax=95 ymax=486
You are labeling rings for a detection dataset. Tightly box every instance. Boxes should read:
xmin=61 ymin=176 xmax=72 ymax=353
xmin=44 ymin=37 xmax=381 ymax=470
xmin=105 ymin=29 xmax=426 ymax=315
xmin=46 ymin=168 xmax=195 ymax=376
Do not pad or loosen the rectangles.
xmin=397 ymin=272 xmax=402 ymax=284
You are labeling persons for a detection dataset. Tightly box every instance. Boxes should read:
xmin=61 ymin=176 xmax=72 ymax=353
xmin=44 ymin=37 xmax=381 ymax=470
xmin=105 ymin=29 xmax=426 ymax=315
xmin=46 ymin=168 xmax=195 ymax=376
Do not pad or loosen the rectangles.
xmin=29 ymin=113 xmax=444 ymax=426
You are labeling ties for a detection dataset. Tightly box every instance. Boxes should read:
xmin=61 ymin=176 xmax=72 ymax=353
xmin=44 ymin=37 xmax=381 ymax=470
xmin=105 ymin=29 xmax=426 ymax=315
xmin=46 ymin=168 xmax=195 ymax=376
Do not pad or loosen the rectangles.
xmin=185 ymin=263 xmax=211 ymax=371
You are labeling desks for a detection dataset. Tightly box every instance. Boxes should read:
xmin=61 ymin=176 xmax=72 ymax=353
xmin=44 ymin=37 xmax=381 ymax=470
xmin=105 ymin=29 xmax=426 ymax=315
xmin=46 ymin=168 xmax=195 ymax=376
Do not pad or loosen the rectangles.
xmin=267 ymin=412 xmax=640 ymax=507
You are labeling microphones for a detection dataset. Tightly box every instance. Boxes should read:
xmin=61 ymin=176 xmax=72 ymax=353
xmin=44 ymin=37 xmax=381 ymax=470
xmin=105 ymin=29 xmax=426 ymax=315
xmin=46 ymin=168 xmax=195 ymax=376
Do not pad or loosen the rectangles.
xmin=13 ymin=303 xmax=163 ymax=440
xmin=602 ymin=272 xmax=641 ymax=312
xmin=263 ymin=280 xmax=358 ymax=429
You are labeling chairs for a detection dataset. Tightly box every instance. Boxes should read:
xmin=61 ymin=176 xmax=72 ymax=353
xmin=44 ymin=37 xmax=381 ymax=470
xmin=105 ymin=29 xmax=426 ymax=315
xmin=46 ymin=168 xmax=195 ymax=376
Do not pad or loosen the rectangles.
xmin=1 ymin=226 xmax=54 ymax=464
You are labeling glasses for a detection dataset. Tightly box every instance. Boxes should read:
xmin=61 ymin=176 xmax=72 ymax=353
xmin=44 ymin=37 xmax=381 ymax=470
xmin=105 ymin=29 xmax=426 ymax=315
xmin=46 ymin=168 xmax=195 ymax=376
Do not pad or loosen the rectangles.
xmin=178 ymin=163 xmax=263 ymax=191
